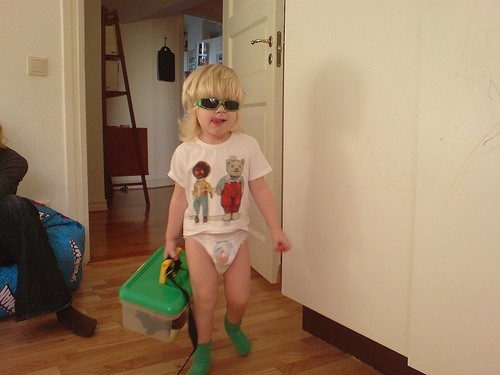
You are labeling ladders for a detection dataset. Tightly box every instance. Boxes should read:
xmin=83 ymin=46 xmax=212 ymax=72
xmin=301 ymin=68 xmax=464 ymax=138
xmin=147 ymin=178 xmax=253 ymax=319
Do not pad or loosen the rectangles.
xmin=101 ymin=7 xmax=152 ymax=213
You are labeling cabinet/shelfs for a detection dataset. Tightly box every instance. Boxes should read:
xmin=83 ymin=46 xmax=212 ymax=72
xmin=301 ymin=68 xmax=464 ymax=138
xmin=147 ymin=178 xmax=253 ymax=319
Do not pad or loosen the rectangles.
xmin=183 ymin=34 xmax=224 ymax=73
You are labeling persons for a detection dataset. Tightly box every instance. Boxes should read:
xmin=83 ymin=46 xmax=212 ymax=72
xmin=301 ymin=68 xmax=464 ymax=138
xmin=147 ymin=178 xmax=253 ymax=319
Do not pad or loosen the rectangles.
xmin=0 ymin=125 xmax=96 ymax=337
xmin=164 ymin=63 xmax=292 ymax=375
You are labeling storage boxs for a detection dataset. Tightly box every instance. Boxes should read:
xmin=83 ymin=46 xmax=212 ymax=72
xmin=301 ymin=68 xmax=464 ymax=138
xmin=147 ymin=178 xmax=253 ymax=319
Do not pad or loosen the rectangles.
xmin=118 ymin=245 xmax=192 ymax=344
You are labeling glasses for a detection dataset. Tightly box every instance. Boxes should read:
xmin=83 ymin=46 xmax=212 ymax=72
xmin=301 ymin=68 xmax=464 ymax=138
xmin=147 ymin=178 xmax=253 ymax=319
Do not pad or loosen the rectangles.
xmin=192 ymin=96 xmax=240 ymax=112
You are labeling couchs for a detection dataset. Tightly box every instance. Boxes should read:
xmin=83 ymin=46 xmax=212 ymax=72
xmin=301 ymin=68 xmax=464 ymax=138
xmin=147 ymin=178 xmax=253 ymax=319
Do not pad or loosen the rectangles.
xmin=0 ymin=200 xmax=85 ymax=322
